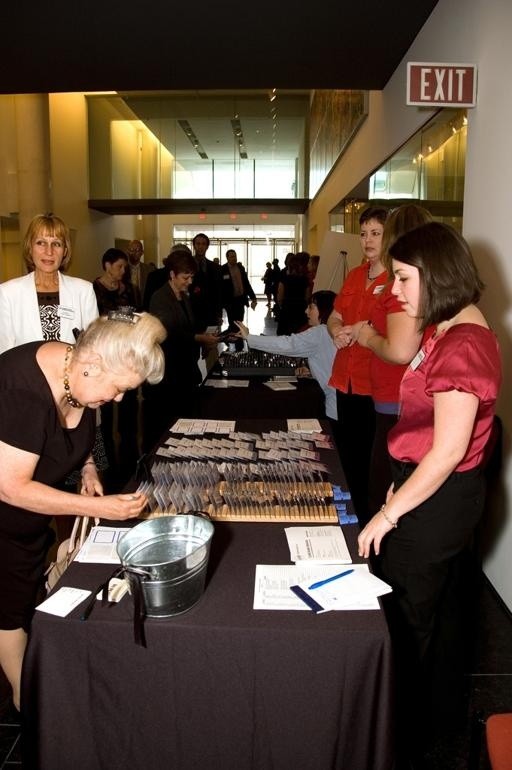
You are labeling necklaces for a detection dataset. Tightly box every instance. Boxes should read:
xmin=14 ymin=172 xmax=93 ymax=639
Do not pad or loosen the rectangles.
xmin=63 ymin=345 xmax=84 ymax=408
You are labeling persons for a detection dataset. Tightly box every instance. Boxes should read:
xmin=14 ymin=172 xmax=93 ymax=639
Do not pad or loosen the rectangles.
xmin=220 ymin=249 xmax=336 ymax=420
xmin=1 ymin=214 xmax=100 ymax=355
xmin=93 ymin=233 xmax=222 ymax=438
xmin=328 ymin=203 xmax=433 ymax=501
xmin=358 ymin=222 xmax=503 ymax=642
xmin=0 ymin=306 xmax=167 ymax=714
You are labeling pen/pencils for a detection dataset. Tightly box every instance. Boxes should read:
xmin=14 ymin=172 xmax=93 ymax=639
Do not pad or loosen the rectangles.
xmin=309 ymin=568 xmax=353 ymax=590
xmin=128 ymin=495 xmax=139 ymax=502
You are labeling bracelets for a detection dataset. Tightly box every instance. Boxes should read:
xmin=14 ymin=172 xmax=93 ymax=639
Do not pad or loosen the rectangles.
xmin=83 ymin=461 xmax=96 ymax=466
xmin=379 ymin=504 xmax=398 ymax=529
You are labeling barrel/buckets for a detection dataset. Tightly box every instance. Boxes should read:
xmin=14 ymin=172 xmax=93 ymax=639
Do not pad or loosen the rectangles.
xmin=115 ymin=511 xmax=215 ymax=619
